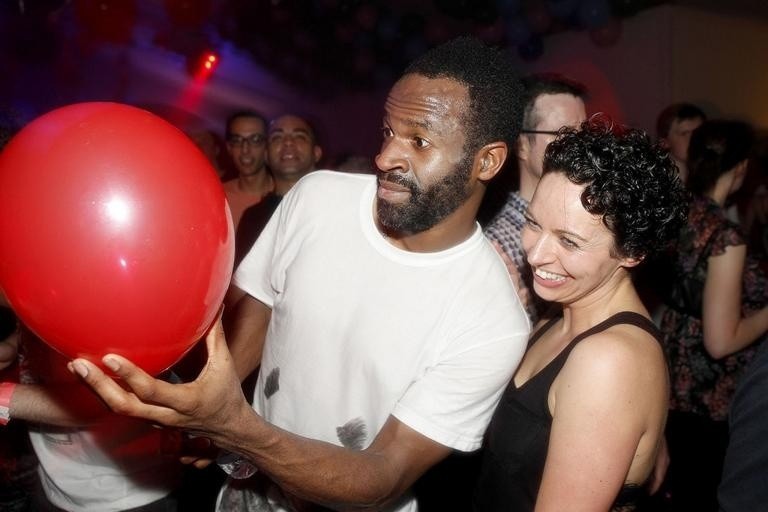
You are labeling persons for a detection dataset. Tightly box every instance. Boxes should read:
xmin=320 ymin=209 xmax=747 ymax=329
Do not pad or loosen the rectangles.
xmin=66 ymin=32 xmax=534 ymax=511
xmin=1 ymin=103 xmax=768 ymax=512
xmin=484 ymin=75 xmax=592 ymax=326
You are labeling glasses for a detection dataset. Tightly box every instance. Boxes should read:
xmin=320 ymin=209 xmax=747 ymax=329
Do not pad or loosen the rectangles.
xmin=229 ymin=133 xmax=263 ymax=148
